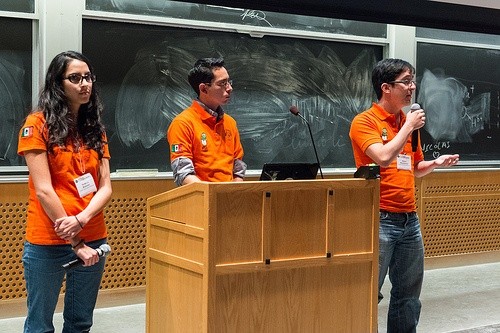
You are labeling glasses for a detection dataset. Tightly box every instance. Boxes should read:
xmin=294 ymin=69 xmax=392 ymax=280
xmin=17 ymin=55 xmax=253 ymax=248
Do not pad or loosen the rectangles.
xmin=60 ymin=73 xmax=97 ymax=85
xmin=204 ymin=79 xmax=234 ymax=87
xmin=387 ymin=79 xmax=418 ymax=87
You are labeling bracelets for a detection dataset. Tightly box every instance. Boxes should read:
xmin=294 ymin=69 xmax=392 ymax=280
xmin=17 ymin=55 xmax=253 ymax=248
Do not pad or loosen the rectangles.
xmin=75 ymin=216 xmax=84 ymax=229
xmin=73 ymin=240 xmax=83 ymax=249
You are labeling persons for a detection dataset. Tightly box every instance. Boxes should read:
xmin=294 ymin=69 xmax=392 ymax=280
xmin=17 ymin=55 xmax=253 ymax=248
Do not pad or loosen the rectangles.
xmin=349 ymin=58 xmax=459 ymax=333
xmin=18 ymin=51 xmax=112 ymax=333
xmin=167 ymin=58 xmax=247 ymax=186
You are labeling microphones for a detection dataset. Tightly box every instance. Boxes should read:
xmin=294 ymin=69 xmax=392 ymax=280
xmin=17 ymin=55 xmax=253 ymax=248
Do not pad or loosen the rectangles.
xmin=410 ymin=103 xmax=421 ymax=152
xmin=290 ymin=105 xmax=324 ymax=179
xmin=62 ymin=243 xmax=111 ymax=271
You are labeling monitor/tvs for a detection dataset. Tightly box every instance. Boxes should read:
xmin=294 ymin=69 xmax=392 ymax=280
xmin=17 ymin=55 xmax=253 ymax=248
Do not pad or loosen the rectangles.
xmin=258 ymin=162 xmax=319 ymax=180
